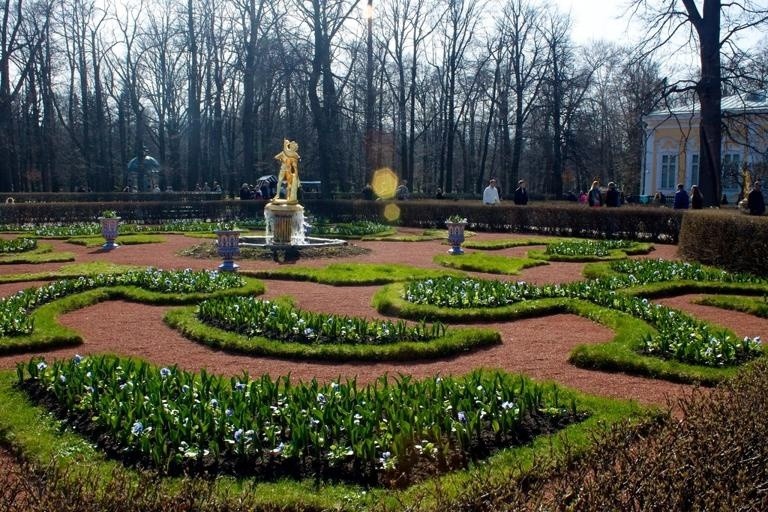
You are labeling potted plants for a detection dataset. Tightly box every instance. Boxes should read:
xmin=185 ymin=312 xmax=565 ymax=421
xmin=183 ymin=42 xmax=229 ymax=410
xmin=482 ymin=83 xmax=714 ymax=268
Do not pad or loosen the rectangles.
xmin=96 ymin=208 xmax=122 ymax=250
xmin=212 ymin=217 xmax=249 ymax=273
xmin=445 ymin=214 xmax=467 ymax=255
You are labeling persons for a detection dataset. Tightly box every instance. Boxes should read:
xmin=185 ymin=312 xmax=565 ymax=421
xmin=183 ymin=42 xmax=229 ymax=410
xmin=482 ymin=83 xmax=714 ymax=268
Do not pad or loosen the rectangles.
xmin=436 ymin=187 xmax=443 ymax=199
xmin=674 ymin=184 xmax=689 ymax=208
xmin=652 ymin=192 xmax=666 ymax=204
xmin=721 ymin=194 xmax=728 ymax=205
xmin=483 ymin=178 xmax=500 ymax=206
xmin=747 ymin=181 xmax=765 ymax=215
xmin=580 ymin=180 xmax=620 ymax=207
xmin=513 ymin=180 xmax=528 ymax=205
xmin=240 ymin=182 xmax=304 ymax=200
xmin=362 ymin=184 xmax=374 ymax=200
xmin=396 ymin=180 xmax=409 ymax=200
xmin=273 ymin=138 xmax=300 ymax=201
xmin=124 ymin=180 xmax=222 ymax=192
xmin=691 ymin=185 xmax=704 ymax=209
